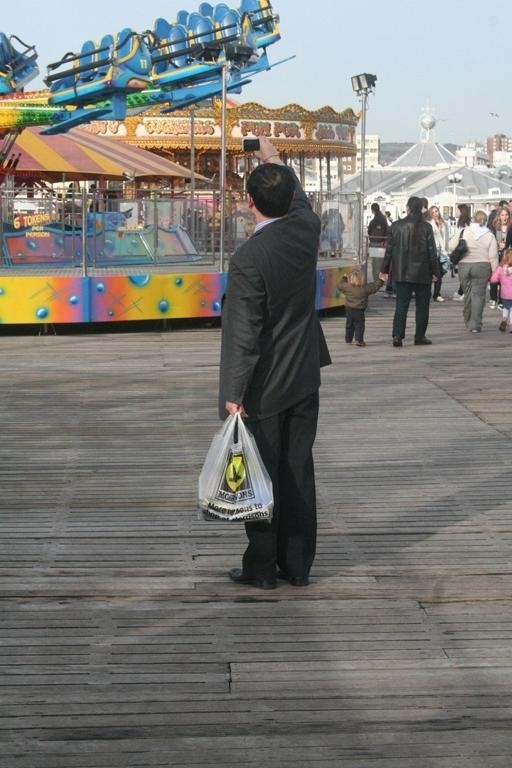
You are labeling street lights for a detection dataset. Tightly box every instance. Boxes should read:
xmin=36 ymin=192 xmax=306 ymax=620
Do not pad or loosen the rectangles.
xmin=449 ymin=227 xmax=468 ymax=265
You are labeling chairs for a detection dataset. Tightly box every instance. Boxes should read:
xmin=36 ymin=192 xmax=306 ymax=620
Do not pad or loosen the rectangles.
xmin=435 ymin=291 xmax=465 ymax=302
xmin=354 ymin=340 xmax=366 ymax=346
xmin=489 ymin=300 xmax=504 ymax=311
xmin=499 ymin=320 xmax=508 ymax=332
xmin=384 ymin=292 xmax=397 ymax=299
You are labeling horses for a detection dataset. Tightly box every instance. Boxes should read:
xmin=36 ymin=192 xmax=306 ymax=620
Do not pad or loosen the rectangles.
xmin=243 ymin=138 xmax=260 ymax=152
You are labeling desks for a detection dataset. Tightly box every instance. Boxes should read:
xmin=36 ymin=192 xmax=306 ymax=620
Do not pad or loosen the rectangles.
xmin=187 ymin=38 xmax=261 ymax=273
xmin=445 ymin=171 xmax=463 ymax=218
xmin=348 ymin=71 xmax=376 ymax=262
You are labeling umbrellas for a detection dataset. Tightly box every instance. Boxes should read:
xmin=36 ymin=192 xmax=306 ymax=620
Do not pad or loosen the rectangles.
xmin=414 ymin=337 xmax=432 ymax=345
xmin=229 ymin=566 xmax=279 ymax=591
xmin=392 ymin=336 xmax=403 ymax=346
xmin=274 ymin=567 xmax=311 ymax=587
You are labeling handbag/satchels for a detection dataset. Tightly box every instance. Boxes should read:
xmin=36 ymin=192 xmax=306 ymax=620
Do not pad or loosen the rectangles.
xmin=261 ymin=153 xmax=280 ymax=161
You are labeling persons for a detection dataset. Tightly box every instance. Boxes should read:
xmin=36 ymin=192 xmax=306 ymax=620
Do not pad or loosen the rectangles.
xmin=89 ymin=185 xmax=100 ymax=213
xmin=335 ymin=195 xmax=512 ymax=347
xmin=219 ymin=138 xmax=331 ymax=593
xmin=65 ymin=183 xmax=74 ymax=203
